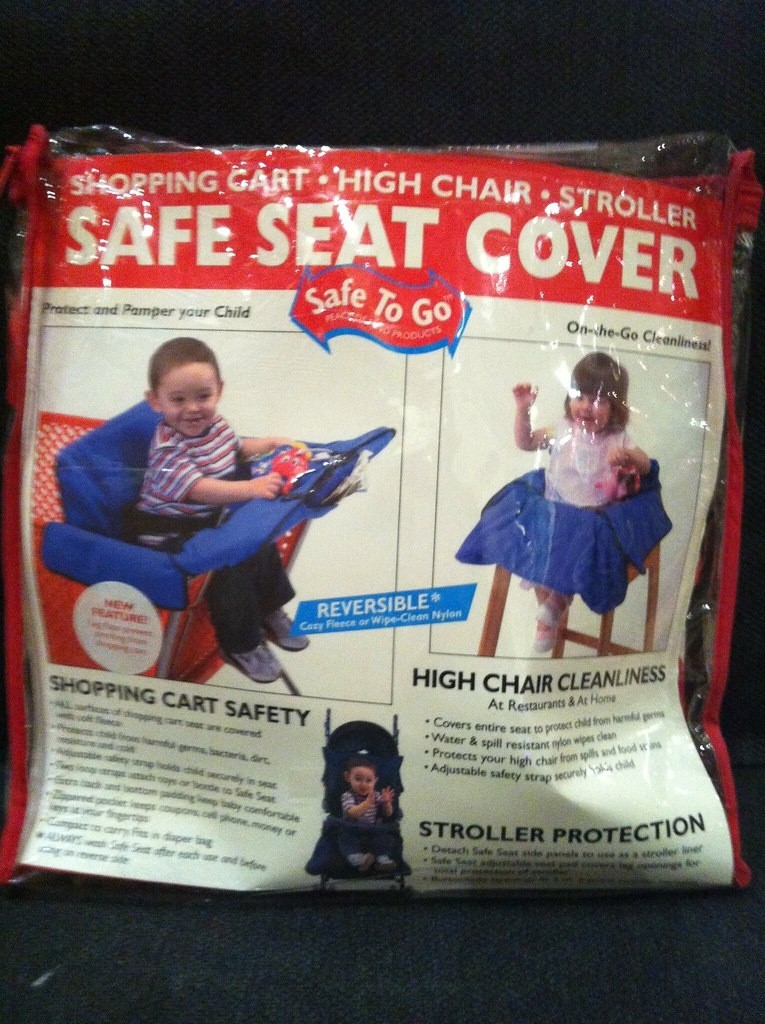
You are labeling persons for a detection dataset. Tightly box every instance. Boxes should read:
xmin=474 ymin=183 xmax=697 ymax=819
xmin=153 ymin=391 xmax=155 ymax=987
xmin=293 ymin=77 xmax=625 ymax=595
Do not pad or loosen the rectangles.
xmin=333 ymin=757 xmax=397 ymax=874
xmin=514 ymin=353 xmax=651 ymax=654
xmin=135 ymin=337 xmax=309 ymax=681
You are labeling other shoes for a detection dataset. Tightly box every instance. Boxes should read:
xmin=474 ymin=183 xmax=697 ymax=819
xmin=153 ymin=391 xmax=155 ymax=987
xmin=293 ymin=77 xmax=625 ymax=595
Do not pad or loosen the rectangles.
xmin=537 ymin=587 xmax=574 ymax=625
xmin=220 ymin=643 xmax=283 ymax=684
xmin=535 ymin=617 xmax=558 ymax=652
xmin=262 ymin=606 xmax=309 ymax=652
xmin=351 ymin=852 xmax=374 ymax=871
xmin=377 ymin=855 xmax=397 ymax=873
